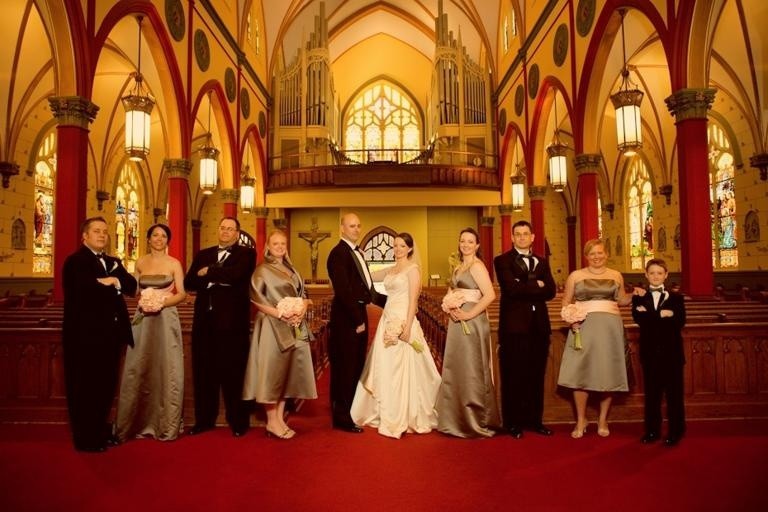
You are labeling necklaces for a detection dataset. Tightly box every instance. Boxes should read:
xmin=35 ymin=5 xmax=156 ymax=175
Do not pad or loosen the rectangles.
xmin=588 ymin=267 xmax=608 ymax=275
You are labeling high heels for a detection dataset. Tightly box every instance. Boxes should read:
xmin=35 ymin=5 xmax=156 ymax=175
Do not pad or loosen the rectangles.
xmin=265 ymin=420 xmax=296 ymax=440
xmin=595 ymin=416 xmax=611 ymax=437
xmin=570 ymin=417 xmax=588 ymax=439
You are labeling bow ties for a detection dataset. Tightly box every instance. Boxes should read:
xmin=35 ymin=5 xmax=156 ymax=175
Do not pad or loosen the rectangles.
xmin=648 ymin=286 xmax=663 ymax=293
xmin=95 ymin=253 xmax=106 ymax=259
xmin=353 ymin=245 xmax=360 ymax=252
xmin=217 ymin=246 xmax=231 ymax=253
xmin=519 ymin=252 xmax=532 ymax=261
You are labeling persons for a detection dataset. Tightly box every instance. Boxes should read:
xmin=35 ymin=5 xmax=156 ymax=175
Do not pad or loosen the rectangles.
xmin=112 ymin=223 xmax=185 ymax=442
xmin=350 ymin=232 xmax=441 ymax=440
xmin=630 ymin=258 xmax=686 ymax=446
xmin=326 ymin=213 xmax=388 ymax=434
xmin=494 ymin=219 xmax=557 ymax=438
xmin=300 ymin=234 xmax=326 ymax=272
xmin=557 ymin=238 xmax=647 ymax=441
xmin=182 ymin=217 xmax=257 ymax=437
xmin=61 ymin=217 xmax=138 ymax=451
xmin=432 ymin=227 xmax=499 ymax=439
xmin=241 ymin=229 xmax=319 ymax=439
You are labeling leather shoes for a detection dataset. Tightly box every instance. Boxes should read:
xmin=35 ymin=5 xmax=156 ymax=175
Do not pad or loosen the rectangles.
xmin=332 ymin=420 xmax=363 ymax=433
xmin=665 ymin=432 xmax=685 ymax=445
xmin=640 ymin=430 xmax=661 ymax=444
xmin=74 ymin=438 xmax=106 ymax=453
xmin=232 ymin=423 xmax=248 ymax=436
xmin=506 ymin=426 xmax=524 ymax=439
xmin=529 ymin=423 xmax=552 ymax=435
xmin=188 ymin=422 xmax=216 ymax=434
xmin=94 ymin=429 xmax=121 ymax=446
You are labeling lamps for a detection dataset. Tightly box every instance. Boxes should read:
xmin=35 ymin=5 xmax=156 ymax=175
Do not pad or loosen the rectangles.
xmin=510 ymin=130 xmax=526 ymax=212
xmin=609 ymin=9 xmax=644 ymax=155
xmin=197 ymin=90 xmax=221 ymax=194
xmin=546 ymin=87 xmax=570 ymax=193
xmin=240 ymin=137 xmax=257 ymax=214
xmin=120 ymin=16 xmax=156 ymax=161
